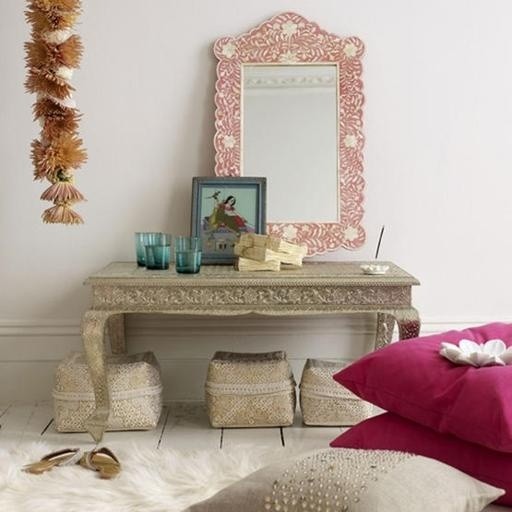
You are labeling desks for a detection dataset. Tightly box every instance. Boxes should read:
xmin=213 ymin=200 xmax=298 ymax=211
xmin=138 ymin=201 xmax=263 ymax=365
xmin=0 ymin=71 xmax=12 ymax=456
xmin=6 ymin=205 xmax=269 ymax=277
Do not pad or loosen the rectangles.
xmin=77 ymin=260 xmax=421 ymax=442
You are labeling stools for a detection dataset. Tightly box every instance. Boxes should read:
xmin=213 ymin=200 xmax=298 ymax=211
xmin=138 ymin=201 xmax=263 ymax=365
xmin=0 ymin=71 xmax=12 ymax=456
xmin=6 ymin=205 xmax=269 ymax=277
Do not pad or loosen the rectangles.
xmin=50 ymin=349 xmax=163 ymax=432
xmin=204 ymin=348 xmax=297 ymax=429
xmin=298 ymin=357 xmax=387 ymax=428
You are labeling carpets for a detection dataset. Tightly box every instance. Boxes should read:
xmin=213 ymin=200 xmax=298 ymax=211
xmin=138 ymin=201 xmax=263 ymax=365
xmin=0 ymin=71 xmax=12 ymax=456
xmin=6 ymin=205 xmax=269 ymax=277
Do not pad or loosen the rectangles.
xmin=0 ymin=434 xmax=272 ymax=512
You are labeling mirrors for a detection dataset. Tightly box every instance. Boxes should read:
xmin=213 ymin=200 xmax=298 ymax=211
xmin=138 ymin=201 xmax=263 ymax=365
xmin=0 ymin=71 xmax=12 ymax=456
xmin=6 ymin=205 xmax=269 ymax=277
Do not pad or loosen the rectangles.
xmin=212 ymin=11 xmax=367 ymax=259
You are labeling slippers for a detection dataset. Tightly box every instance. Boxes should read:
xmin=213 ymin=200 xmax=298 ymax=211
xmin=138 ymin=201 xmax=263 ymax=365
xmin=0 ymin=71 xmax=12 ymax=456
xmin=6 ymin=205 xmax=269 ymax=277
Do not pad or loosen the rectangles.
xmin=76 ymin=447 xmax=122 ymax=481
xmin=20 ymin=445 xmax=87 ymax=476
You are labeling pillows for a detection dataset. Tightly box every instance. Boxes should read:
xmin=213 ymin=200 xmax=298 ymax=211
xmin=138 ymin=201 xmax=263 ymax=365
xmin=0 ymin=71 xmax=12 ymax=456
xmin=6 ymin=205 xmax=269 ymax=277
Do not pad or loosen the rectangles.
xmin=332 ymin=321 xmax=512 ymax=455
xmin=186 ymin=447 xmax=507 ymax=511
xmin=328 ymin=411 xmax=512 ymax=507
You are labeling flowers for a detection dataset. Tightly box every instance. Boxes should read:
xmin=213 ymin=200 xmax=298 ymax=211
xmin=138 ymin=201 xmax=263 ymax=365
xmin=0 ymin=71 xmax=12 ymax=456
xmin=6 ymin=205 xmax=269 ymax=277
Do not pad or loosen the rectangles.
xmin=38 ymin=164 xmax=87 ymax=226
xmin=437 ymin=335 xmax=512 ymax=368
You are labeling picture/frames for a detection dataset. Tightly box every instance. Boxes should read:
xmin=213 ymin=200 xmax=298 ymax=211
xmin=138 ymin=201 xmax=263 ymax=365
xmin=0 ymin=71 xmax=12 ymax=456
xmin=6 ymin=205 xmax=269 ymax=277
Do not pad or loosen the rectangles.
xmin=189 ymin=177 xmax=266 ymax=264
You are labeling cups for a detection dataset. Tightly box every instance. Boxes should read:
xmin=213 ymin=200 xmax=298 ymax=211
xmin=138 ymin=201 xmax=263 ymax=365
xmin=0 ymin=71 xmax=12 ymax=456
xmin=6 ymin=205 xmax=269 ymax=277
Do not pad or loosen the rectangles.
xmin=174 ymin=236 xmax=202 ymax=274
xmin=134 ymin=231 xmax=146 ymax=267
xmin=143 ymin=232 xmax=172 ymax=270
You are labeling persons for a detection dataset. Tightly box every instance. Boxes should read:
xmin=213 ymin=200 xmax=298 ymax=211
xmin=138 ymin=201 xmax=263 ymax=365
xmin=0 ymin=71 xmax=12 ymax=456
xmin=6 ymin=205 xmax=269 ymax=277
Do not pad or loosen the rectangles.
xmin=217 ymin=196 xmax=254 ymax=232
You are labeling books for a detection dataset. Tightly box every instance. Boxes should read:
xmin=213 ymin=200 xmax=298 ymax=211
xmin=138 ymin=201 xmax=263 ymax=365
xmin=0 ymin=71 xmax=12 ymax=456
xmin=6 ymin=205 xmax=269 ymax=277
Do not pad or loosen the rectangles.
xmin=231 ymin=232 xmax=307 ymax=272
